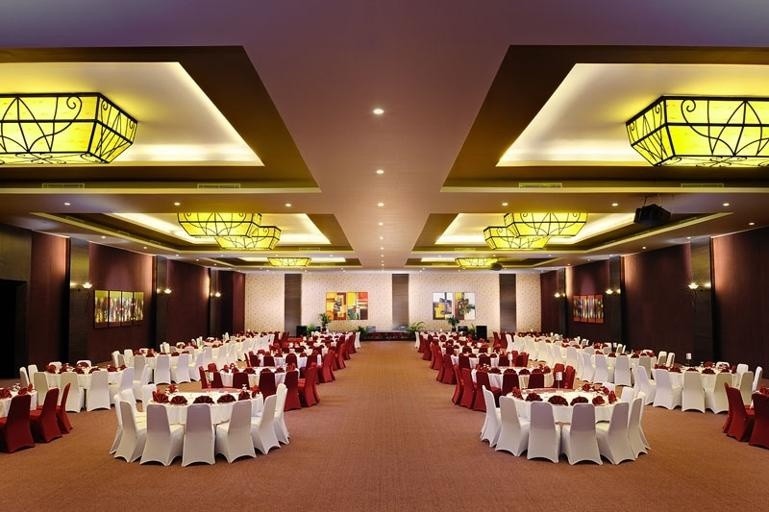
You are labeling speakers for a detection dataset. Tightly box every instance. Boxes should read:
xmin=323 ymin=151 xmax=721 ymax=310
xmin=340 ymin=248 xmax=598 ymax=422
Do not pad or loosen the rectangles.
xmin=634 ymin=204 xmax=670 ymax=226
